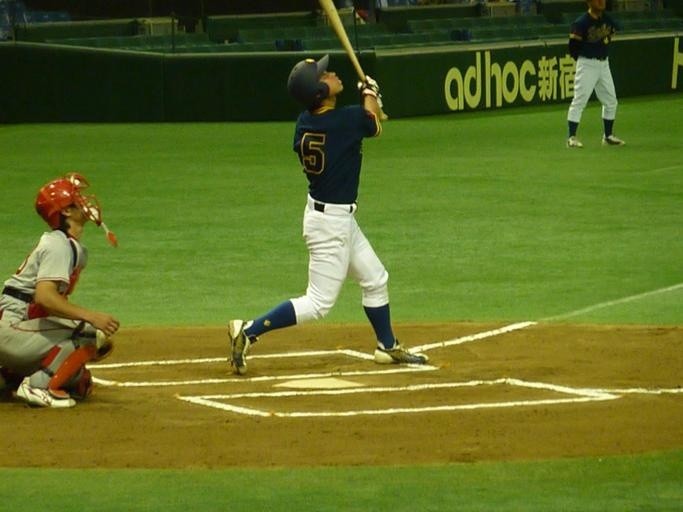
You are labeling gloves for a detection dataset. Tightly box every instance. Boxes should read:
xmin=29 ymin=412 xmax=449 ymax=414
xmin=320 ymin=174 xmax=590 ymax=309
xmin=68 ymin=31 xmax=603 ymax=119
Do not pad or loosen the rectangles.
xmin=357 ymin=76 xmax=383 ymax=109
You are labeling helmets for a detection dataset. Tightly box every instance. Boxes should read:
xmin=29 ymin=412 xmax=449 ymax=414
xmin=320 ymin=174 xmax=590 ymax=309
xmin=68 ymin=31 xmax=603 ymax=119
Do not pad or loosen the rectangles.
xmin=287 ymin=53 xmax=330 ymax=107
xmin=36 ymin=172 xmax=101 ymax=229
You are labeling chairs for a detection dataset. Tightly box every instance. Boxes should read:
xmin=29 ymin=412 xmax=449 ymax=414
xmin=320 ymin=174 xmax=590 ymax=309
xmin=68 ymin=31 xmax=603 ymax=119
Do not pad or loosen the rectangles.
xmin=128 ymin=11 xmax=681 ymax=52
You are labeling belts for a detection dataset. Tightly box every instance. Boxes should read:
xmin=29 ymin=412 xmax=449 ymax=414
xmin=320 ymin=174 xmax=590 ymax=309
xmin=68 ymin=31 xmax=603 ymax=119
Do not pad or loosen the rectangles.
xmin=2 ymin=287 xmax=34 ymax=303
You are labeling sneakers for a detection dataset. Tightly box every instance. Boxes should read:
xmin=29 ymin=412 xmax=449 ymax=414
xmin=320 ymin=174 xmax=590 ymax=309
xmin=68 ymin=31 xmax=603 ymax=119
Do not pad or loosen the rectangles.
xmin=12 ymin=376 xmax=77 ymax=408
xmin=567 ymin=136 xmax=584 ymax=148
xmin=227 ymin=319 xmax=259 ymax=376
xmin=373 ymin=339 xmax=431 ymax=365
xmin=601 ymin=135 xmax=625 ymax=146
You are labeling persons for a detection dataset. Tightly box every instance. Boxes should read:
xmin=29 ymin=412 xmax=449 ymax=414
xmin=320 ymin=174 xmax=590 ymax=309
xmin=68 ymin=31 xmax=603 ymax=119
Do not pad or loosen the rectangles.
xmin=565 ymin=0 xmax=625 ymax=151
xmin=226 ymin=55 xmax=429 ymax=376
xmin=1 ymin=174 xmax=120 ymax=409
